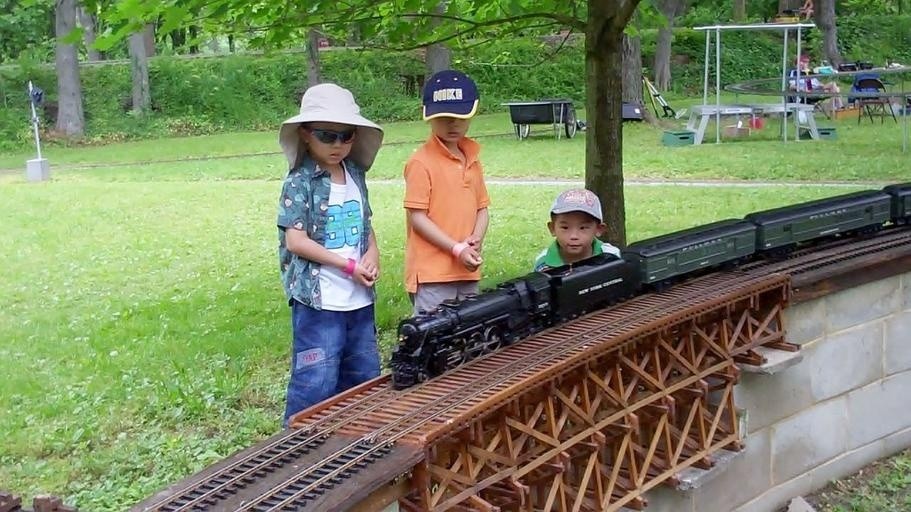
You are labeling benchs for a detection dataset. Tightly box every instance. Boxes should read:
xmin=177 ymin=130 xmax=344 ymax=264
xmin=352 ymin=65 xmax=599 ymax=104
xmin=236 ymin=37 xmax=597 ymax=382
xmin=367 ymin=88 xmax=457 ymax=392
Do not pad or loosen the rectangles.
xmin=501 ymin=96 xmax=578 ymax=141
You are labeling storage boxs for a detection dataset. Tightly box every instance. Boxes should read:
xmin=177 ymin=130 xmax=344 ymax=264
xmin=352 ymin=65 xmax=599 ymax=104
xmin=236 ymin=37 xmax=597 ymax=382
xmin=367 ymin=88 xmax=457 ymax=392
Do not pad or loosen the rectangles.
xmin=798 ymin=126 xmax=838 ymax=143
xmin=662 ymin=128 xmax=696 ymax=149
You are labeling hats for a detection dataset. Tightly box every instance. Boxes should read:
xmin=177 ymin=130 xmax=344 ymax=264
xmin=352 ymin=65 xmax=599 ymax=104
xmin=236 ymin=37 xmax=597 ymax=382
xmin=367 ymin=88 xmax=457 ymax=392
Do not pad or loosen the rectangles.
xmin=550 ymin=188 xmax=603 ymax=223
xmin=278 ymin=83 xmax=384 ymax=177
xmin=422 ymin=71 xmax=481 ymax=122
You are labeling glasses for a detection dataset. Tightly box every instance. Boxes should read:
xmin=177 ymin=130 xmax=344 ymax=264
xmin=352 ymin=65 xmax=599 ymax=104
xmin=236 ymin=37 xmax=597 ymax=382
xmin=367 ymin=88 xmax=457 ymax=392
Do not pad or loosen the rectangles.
xmin=302 ymin=125 xmax=357 ymax=144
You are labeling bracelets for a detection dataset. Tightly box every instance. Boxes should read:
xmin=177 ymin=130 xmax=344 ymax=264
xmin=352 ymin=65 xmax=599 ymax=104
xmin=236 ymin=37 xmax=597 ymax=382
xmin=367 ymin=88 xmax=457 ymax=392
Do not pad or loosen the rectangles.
xmin=451 ymin=242 xmax=470 ymax=259
xmin=342 ymin=258 xmax=356 ymax=275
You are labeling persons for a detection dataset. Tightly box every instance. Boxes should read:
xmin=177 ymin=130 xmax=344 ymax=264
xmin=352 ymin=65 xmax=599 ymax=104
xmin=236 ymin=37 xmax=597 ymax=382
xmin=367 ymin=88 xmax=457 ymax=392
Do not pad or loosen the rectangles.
xmin=787 ymin=54 xmax=845 ymax=111
xmin=401 ymin=67 xmax=489 ymax=318
xmin=796 ymin=0 xmax=815 ymax=21
xmin=533 ymin=186 xmax=622 ymax=273
xmin=274 ymin=82 xmax=386 ymax=433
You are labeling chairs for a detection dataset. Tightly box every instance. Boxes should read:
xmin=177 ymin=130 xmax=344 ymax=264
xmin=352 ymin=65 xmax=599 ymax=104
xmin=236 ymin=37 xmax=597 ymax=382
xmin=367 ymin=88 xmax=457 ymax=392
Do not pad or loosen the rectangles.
xmin=854 ymin=77 xmax=899 ymax=123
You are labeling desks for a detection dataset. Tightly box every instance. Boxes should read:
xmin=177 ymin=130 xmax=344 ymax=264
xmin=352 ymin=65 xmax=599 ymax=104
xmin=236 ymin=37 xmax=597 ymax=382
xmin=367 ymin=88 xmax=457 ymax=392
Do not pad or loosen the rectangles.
xmin=684 ymin=102 xmax=821 ymax=143
